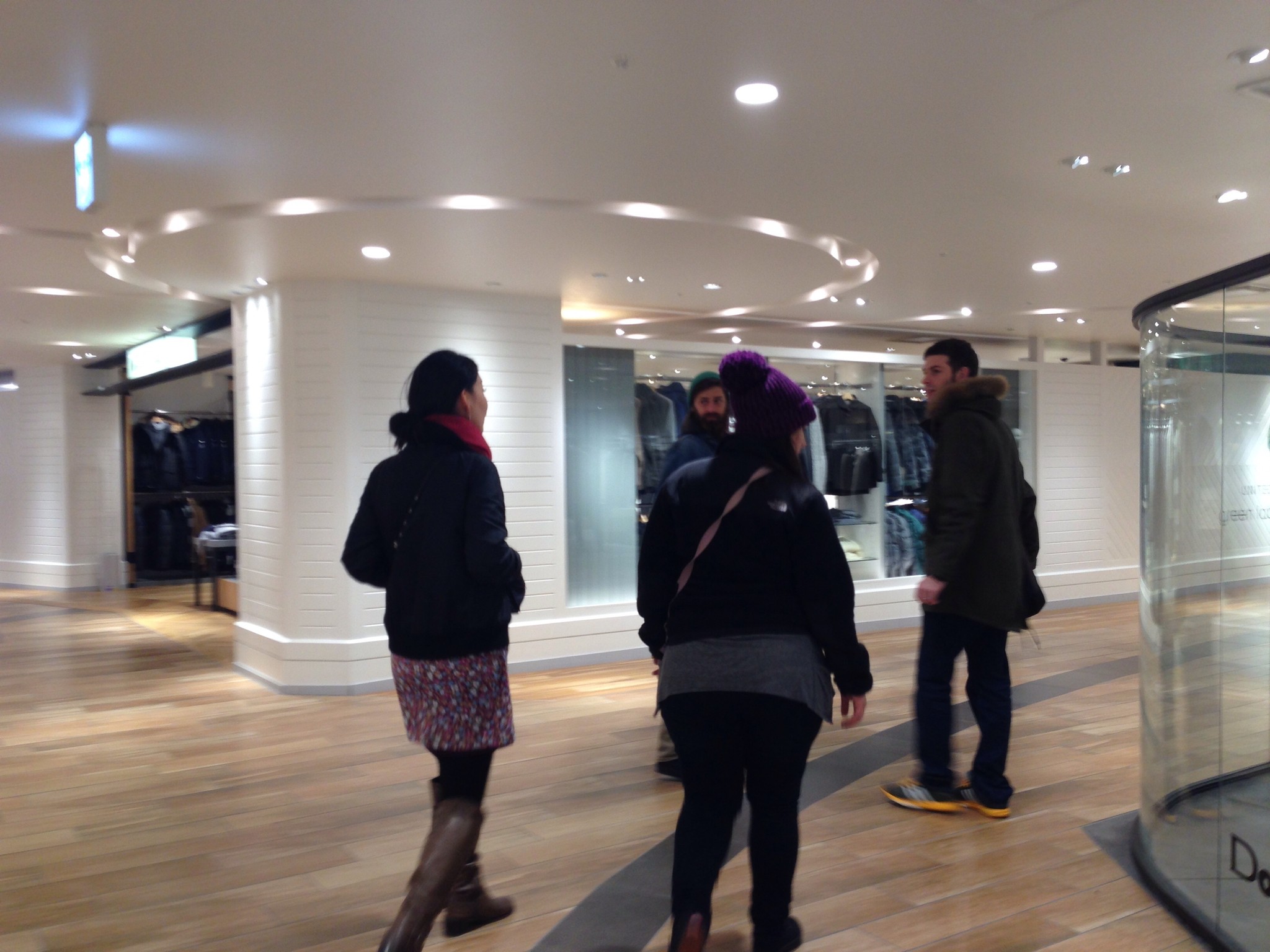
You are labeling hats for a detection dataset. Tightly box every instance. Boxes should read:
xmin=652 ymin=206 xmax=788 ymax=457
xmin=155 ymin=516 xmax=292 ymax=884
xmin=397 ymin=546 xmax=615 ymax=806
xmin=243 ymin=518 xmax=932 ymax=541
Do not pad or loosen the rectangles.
xmin=688 ymin=371 xmax=731 ymax=408
xmin=719 ymin=350 xmax=817 ymax=439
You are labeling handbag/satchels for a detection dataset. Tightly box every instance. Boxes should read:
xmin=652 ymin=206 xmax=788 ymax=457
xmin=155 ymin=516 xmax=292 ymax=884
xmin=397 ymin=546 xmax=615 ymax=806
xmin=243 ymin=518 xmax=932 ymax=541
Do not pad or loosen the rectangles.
xmin=1021 ymin=545 xmax=1045 ymax=618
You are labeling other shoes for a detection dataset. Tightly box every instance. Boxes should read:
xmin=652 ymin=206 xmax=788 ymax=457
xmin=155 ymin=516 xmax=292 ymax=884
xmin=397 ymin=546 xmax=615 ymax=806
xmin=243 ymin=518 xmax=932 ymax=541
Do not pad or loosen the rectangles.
xmin=669 ymin=899 xmax=712 ymax=952
xmin=752 ymin=916 xmax=802 ymax=952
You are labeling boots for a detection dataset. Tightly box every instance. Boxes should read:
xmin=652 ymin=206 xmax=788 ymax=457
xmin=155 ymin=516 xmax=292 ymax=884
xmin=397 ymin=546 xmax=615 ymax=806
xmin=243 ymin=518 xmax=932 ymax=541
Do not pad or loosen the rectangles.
xmin=376 ymin=798 xmax=485 ymax=952
xmin=429 ymin=775 xmax=515 ymax=938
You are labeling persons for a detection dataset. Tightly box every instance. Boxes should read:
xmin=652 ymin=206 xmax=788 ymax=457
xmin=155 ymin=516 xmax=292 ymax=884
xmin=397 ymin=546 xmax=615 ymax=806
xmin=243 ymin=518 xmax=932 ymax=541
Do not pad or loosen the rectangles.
xmin=655 ymin=372 xmax=727 ymax=780
xmin=342 ymin=350 xmax=526 ymax=951
xmin=880 ymin=338 xmax=1048 ymax=816
xmin=638 ymin=350 xmax=873 ymax=952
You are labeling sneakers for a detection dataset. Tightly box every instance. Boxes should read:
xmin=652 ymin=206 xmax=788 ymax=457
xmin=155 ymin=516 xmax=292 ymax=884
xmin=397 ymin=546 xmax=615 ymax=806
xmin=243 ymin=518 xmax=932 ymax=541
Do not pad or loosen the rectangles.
xmin=955 ymin=773 xmax=1012 ymax=818
xmin=878 ymin=776 xmax=969 ymax=812
